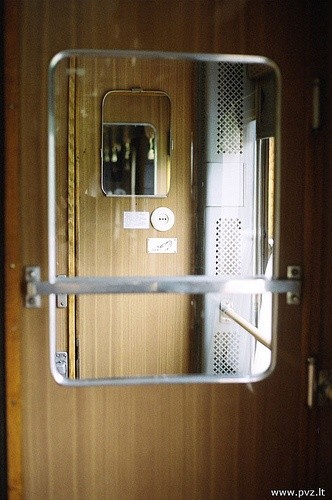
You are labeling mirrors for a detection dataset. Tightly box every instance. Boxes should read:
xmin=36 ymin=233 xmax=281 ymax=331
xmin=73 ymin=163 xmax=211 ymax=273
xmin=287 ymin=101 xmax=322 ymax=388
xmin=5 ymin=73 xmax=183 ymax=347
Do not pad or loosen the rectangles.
xmin=47 ymin=50 xmax=281 ymax=387
xmin=101 ymin=88 xmax=173 ymax=198
xmin=103 ymin=122 xmax=157 ymax=197
